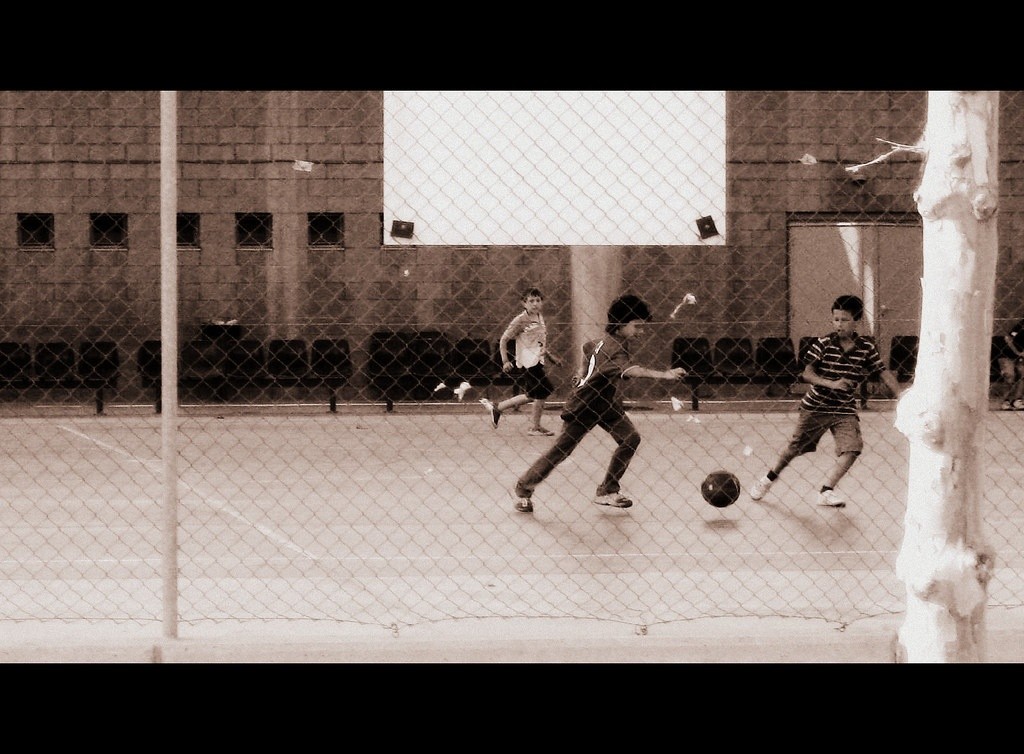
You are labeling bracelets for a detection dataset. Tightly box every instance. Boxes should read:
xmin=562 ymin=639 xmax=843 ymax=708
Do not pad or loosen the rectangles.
xmin=545 ymin=351 xmax=552 ymax=357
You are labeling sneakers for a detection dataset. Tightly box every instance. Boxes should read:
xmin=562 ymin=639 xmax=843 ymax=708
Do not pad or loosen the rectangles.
xmin=513 ymin=492 xmax=534 ymax=514
xmin=750 ymin=477 xmax=773 ymax=500
xmin=817 ymin=489 xmax=846 ymax=507
xmin=594 ymin=489 xmax=633 ymax=508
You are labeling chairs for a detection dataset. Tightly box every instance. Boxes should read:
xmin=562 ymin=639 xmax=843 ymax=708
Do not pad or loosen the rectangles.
xmin=267 ymin=338 xmax=309 ymax=384
xmin=78 ymin=340 xmax=121 ymax=387
xmin=309 ymin=339 xmax=350 ymax=382
xmin=493 ymin=339 xmax=518 ymax=386
xmin=672 ymin=338 xmax=714 ymax=382
xmin=34 ymin=342 xmax=78 ymax=388
xmin=412 ymin=329 xmax=452 ymax=383
xmin=456 ymin=339 xmax=494 ymax=387
xmin=367 ymin=332 xmax=413 ymax=379
xmin=799 ymin=336 xmax=820 ymax=384
xmin=989 ymin=336 xmax=1011 ymax=380
xmin=890 ymin=335 xmax=919 ymax=382
xmin=224 ymin=340 xmax=265 ymax=382
xmin=0 ymin=342 xmax=32 ymax=388
xmin=755 ymin=338 xmax=797 ymax=384
xmin=137 ymin=339 xmax=163 ymax=386
xmin=714 ymin=338 xmax=755 ymax=382
xmin=183 ymin=341 xmax=220 ymax=381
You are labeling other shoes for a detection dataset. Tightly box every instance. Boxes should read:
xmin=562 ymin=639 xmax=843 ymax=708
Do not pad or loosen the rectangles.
xmin=490 ymin=400 xmax=503 ymax=429
xmin=999 ymin=400 xmax=1023 ymax=409
xmin=526 ymin=424 xmax=554 ymax=437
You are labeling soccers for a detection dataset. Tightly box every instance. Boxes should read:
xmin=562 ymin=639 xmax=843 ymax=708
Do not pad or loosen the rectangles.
xmin=700 ymin=470 xmax=741 ymax=507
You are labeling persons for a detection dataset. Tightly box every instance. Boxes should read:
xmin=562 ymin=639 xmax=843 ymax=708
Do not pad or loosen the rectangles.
xmin=997 ymin=319 xmax=1024 ymax=411
xmin=488 ymin=288 xmax=564 ymax=436
xmin=749 ymin=295 xmax=903 ymax=507
xmin=512 ymin=295 xmax=688 ymax=511
